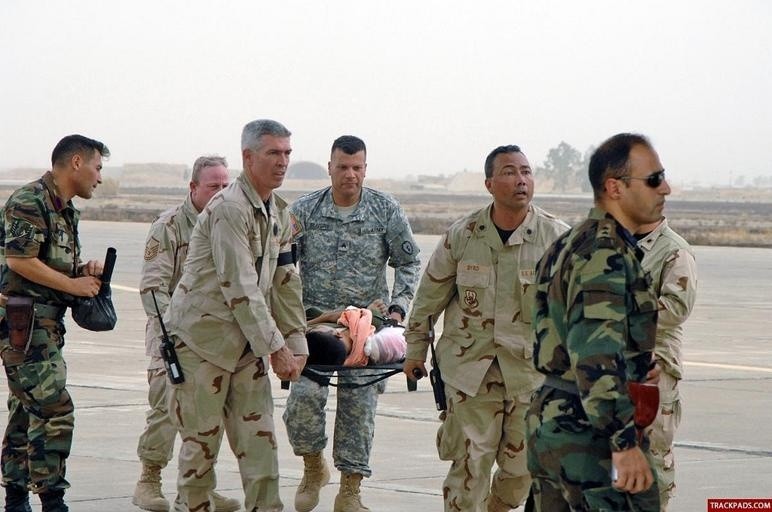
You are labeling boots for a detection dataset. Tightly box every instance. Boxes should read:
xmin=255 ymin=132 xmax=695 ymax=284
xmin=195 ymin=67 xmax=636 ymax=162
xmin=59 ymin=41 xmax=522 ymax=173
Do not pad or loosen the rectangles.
xmin=294 ymin=449 xmax=331 ymax=512
xmin=334 ymin=470 xmax=373 ymax=512
xmin=4 ymin=486 xmax=69 ymax=511
xmin=131 ymin=464 xmax=170 ymax=512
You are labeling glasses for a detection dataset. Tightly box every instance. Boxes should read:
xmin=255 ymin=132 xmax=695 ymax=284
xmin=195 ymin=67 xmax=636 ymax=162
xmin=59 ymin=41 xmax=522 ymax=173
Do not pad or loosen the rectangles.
xmin=615 ymin=172 xmax=665 ymax=190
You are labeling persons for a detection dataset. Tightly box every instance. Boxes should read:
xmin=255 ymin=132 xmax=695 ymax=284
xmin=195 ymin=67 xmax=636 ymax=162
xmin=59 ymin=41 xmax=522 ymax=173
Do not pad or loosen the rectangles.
xmin=632 ymin=213 xmax=702 ymax=512
xmin=518 ymin=130 xmax=673 ymax=512
xmin=302 ymin=297 xmax=408 ymax=371
xmin=279 ymin=134 xmax=423 ymax=511
xmin=402 ymin=143 xmax=574 ymax=512
xmin=0 ymin=132 xmax=114 ymax=512
xmin=128 ymin=153 xmax=243 ymax=512
xmin=159 ymin=116 xmax=311 ymax=512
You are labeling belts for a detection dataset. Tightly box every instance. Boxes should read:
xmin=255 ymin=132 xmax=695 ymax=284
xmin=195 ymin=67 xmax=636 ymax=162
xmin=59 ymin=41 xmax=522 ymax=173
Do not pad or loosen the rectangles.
xmin=543 ymin=375 xmax=580 ymax=397
xmin=0 ymin=294 xmax=60 ymax=321
